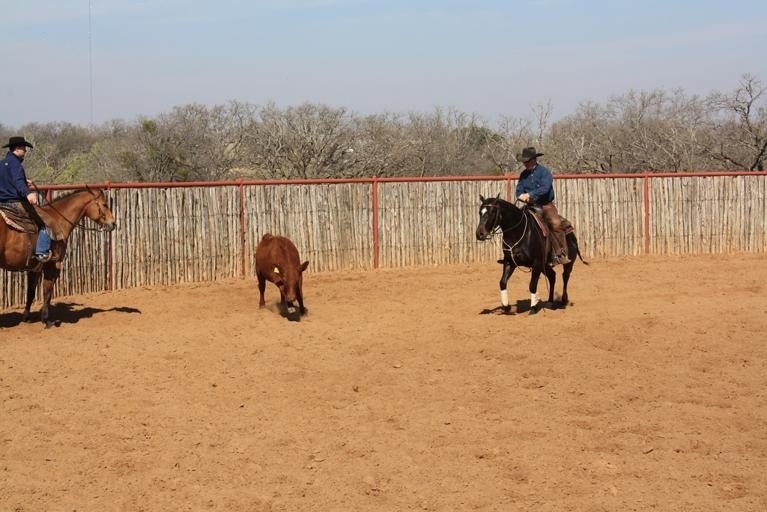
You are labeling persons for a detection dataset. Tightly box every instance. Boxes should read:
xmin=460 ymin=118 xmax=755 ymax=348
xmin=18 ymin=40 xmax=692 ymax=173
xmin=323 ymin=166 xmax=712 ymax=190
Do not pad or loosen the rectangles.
xmin=0 ymin=136 xmax=58 ymax=263
xmin=496 ymin=146 xmax=572 ymax=265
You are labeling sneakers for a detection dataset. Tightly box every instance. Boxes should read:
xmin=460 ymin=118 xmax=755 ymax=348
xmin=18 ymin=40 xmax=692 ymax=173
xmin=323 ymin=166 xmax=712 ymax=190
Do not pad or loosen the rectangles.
xmin=40 ymin=253 xmax=57 ymax=262
xmin=497 ymin=259 xmax=504 ymax=264
xmin=559 ymin=257 xmax=572 ymax=264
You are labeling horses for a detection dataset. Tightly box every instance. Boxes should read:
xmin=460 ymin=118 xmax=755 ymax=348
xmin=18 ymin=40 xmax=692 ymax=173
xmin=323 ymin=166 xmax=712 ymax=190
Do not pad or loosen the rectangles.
xmin=0 ymin=183 xmax=118 ymax=324
xmin=475 ymin=191 xmax=588 ymax=316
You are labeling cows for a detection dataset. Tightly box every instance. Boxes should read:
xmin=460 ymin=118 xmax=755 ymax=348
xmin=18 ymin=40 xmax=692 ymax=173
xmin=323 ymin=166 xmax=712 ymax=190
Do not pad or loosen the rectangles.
xmin=254 ymin=232 xmax=310 ymax=315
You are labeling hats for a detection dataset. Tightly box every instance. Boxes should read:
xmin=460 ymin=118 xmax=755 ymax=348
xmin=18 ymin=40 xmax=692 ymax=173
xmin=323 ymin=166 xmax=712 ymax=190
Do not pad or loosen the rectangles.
xmin=516 ymin=147 xmax=544 ymax=162
xmin=1 ymin=136 xmax=33 ymax=149
